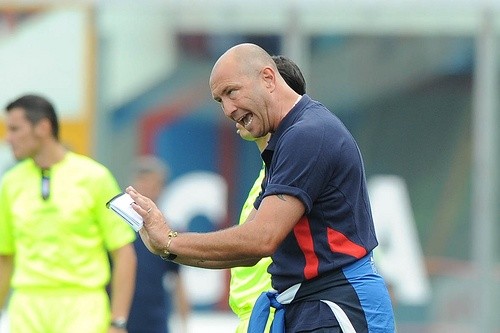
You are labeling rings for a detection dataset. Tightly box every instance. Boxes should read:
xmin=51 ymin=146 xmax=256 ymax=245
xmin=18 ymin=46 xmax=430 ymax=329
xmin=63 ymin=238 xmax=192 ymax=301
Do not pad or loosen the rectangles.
xmin=148 ymin=208 xmax=152 ymax=212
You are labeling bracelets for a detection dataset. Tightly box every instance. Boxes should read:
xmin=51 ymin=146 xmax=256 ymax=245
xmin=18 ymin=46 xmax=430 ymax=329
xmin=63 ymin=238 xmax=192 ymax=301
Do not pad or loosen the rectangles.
xmin=164 ymin=232 xmax=177 ymax=254
xmin=161 ymin=253 xmax=177 ymax=263
xmin=111 ymin=321 xmax=126 ymax=328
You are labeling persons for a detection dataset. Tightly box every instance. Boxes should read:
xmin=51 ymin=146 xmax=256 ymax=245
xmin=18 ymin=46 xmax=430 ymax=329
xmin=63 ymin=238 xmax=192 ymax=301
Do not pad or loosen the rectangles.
xmin=126 ymin=43 xmax=396 ymax=333
xmin=229 ymin=55 xmax=308 ymax=333
xmin=0 ymin=95 xmax=137 ymax=333
xmin=124 ymin=154 xmax=180 ymax=333
